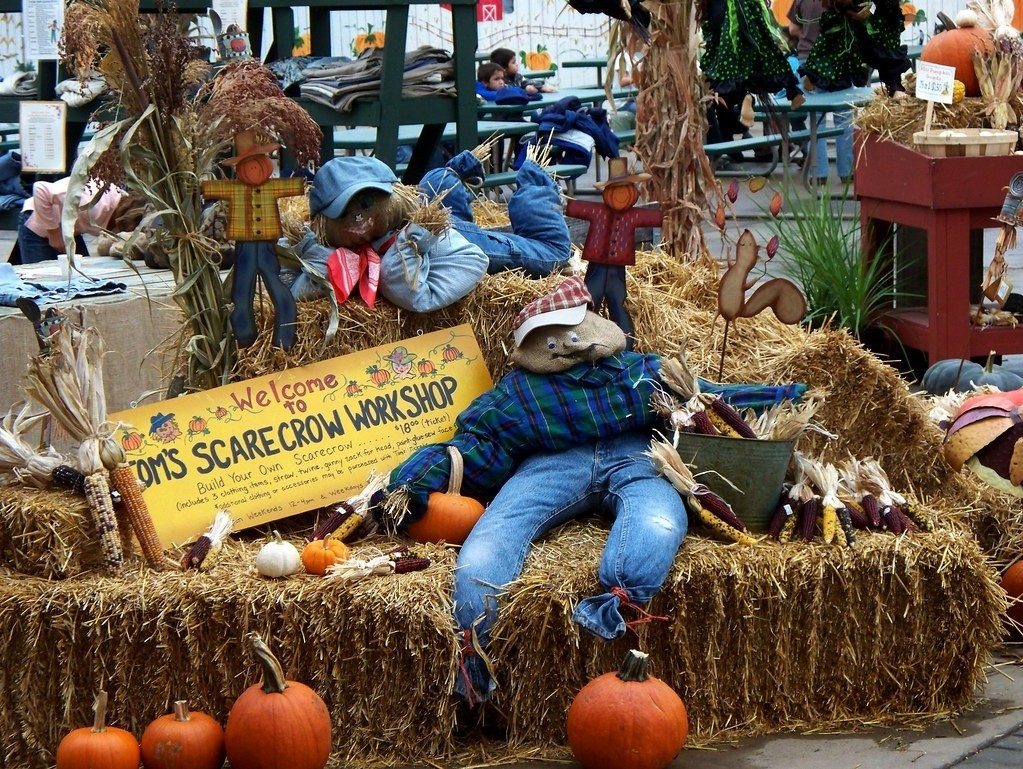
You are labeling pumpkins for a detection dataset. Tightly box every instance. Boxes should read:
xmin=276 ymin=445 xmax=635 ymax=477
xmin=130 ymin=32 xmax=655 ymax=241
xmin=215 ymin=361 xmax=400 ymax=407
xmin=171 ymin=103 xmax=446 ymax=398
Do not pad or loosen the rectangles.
xmin=405 ymin=446 xmax=485 ymax=549
xmin=919 ymin=348 xmax=1023 ymax=396
xmin=302 ymin=533 xmax=349 ymax=575
xmin=920 ymin=7 xmax=997 ymax=97
xmin=231 ymin=38 xmax=246 ymax=52
xmin=566 ymin=649 xmax=689 ymax=769
xmin=1000 ymin=562 xmax=1023 ymax=634
xmin=256 ymin=530 xmax=300 ymax=578
xmin=55 ymin=689 xmax=140 ymax=769
xmin=224 ymin=630 xmax=333 ymax=769
xmin=139 ymin=699 xmax=227 ymax=769
xmin=323 ymin=188 xmax=404 ymax=249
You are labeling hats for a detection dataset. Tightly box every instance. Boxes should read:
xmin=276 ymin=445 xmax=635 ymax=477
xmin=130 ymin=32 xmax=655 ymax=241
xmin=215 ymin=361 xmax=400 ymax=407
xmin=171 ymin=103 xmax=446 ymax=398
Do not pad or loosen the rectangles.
xmin=495 ymin=87 xmax=528 ymax=105
xmin=310 ymin=156 xmax=398 ymax=220
xmin=513 ymin=276 xmax=594 ymax=347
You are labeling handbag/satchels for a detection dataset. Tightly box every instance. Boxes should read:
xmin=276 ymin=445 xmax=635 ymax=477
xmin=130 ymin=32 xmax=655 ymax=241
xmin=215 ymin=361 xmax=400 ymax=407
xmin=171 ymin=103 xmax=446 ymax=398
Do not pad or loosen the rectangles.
xmin=217 ymin=24 xmax=253 ymax=59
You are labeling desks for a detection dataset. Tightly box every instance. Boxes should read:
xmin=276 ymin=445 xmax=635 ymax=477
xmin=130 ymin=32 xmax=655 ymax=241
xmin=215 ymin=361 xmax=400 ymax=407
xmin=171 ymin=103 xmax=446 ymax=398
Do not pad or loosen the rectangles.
xmin=476 ymin=88 xmax=638 ymax=182
xmin=330 ymin=120 xmax=546 ymax=151
xmin=474 ymin=52 xmax=607 ymax=86
xmin=752 ymin=45 xmax=928 ymax=202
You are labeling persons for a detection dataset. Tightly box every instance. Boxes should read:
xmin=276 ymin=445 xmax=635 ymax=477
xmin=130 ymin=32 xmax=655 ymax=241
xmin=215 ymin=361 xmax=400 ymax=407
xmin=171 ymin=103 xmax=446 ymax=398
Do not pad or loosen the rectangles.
xmin=474 ymin=62 xmax=538 ymax=101
xmin=490 ymin=47 xmax=556 ymax=171
xmin=18 ymin=172 xmax=150 ymax=263
xmin=785 ymin=0 xmax=856 ymax=186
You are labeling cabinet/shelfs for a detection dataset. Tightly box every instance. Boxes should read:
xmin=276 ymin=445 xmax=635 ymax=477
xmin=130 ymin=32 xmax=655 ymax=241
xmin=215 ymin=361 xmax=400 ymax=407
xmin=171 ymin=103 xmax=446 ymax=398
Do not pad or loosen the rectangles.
xmin=852 ymin=127 xmax=1023 ymax=366
xmin=246 ymin=0 xmax=478 ymax=178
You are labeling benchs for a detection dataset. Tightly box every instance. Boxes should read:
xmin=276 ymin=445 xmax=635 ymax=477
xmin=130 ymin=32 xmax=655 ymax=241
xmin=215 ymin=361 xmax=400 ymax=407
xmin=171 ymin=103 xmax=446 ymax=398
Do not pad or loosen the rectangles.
xmin=484 ymin=163 xmax=588 ymax=187
xmin=616 ymin=129 xmax=639 ymax=144
xmin=692 ymin=127 xmax=844 ymax=179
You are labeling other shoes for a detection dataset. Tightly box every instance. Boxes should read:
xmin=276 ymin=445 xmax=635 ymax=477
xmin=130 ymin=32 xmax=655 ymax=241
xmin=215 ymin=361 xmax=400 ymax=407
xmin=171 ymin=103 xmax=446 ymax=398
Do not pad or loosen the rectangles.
xmin=809 ymin=176 xmax=828 ymax=186
xmin=841 ymin=175 xmax=855 ymax=184
xmin=778 ymin=142 xmax=795 ymax=152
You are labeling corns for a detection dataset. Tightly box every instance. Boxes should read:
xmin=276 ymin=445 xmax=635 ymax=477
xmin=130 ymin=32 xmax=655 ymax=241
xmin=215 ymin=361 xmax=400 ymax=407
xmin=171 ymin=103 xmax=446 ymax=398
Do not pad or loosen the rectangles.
xmin=689 ymin=401 xmax=923 ymax=546
xmin=301 ymin=502 xmax=365 ymax=544
xmin=51 ymin=463 xmax=166 ymax=576
xmin=382 ymin=545 xmax=431 ymax=573
xmin=999 ymin=34 xmax=1023 ymax=59
xmin=180 ymin=536 xmax=220 ymax=571
